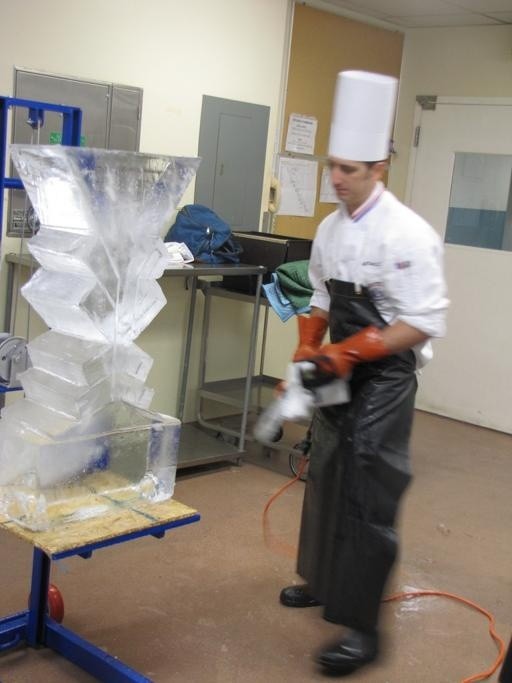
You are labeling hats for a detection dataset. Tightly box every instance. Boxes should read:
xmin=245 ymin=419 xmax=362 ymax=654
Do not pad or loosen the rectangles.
xmin=328 ymin=70 xmax=400 ymax=162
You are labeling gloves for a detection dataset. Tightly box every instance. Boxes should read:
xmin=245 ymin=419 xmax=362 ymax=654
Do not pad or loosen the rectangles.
xmin=313 ymin=323 xmax=390 ymax=380
xmin=272 ymin=314 xmax=328 ymax=398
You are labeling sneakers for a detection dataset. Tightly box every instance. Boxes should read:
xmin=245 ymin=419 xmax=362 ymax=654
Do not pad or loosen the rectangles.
xmin=311 ymin=629 xmax=379 ymax=673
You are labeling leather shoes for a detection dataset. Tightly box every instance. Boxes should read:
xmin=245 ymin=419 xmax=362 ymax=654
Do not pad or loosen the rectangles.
xmin=279 ymin=582 xmax=326 ymax=608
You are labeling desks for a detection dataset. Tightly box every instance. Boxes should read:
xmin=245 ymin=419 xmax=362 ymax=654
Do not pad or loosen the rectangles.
xmin=1 ymin=469 xmax=202 ymax=683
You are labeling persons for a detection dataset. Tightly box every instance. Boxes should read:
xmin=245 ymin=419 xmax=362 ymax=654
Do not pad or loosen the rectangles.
xmin=280 ymin=156 xmax=450 ymax=668
